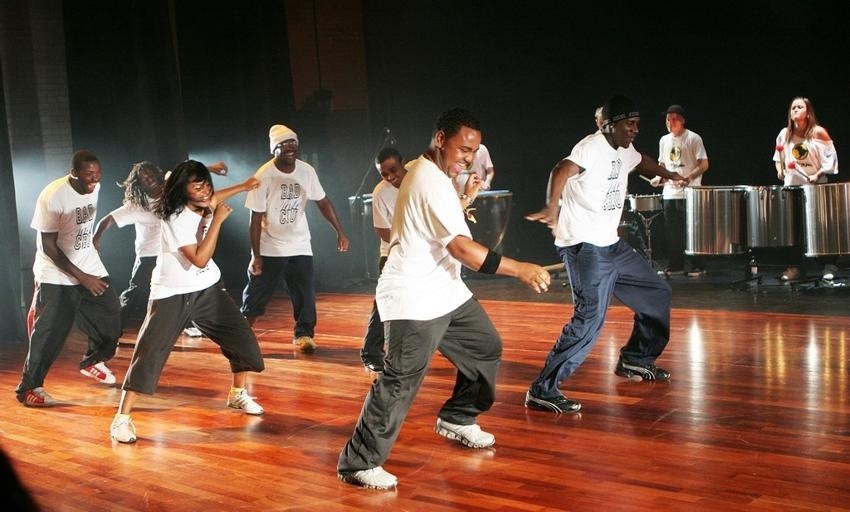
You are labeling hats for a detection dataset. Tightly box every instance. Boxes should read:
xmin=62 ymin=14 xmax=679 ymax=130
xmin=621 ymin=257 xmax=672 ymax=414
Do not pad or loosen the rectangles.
xmin=269 ymin=125 xmax=299 ymax=154
xmin=602 ymin=95 xmax=639 ymax=125
xmin=666 ymin=105 xmax=683 ymax=113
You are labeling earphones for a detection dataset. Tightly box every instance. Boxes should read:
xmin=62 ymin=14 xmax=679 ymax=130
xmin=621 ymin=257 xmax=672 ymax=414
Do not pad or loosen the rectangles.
xmin=610 ymin=125 xmax=613 ymax=129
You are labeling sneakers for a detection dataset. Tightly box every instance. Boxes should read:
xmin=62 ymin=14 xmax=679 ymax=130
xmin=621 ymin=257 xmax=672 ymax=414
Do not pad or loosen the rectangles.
xmin=525 ymin=391 xmax=581 ymax=413
xmin=338 ymin=465 xmax=398 ymax=490
xmin=80 ymin=362 xmax=115 ymax=383
xmin=24 ymin=387 xmax=55 ymax=406
xmin=436 ymin=417 xmax=496 ymax=448
xmin=615 ymin=355 xmax=670 ymax=380
xmin=227 ymin=390 xmax=263 ymax=414
xmin=112 ymin=422 xmax=136 ymax=443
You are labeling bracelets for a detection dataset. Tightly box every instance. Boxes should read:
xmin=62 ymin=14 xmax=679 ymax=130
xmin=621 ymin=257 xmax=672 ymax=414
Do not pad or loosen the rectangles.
xmin=479 ymin=248 xmax=503 ymax=278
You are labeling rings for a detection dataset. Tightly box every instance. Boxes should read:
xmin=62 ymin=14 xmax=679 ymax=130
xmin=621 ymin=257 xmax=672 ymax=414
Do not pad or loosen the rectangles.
xmin=540 ymin=283 xmax=546 ymax=288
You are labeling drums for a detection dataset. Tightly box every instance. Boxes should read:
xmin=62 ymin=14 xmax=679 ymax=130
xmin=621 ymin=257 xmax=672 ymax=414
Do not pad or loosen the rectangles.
xmin=684 ymin=184 xmax=747 ymax=257
xmin=744 ymin=187 xmax=798 ymax=249
xmin=627 ymin=194 xmax=664 ymax=213
xmin=466 ymin=190 xmax=513 ymax=253
xmin=362 ymin=200 xmax=383 ymax=280
xmin=349 ymin=194 xmax=372 ymax=231
xmin=802 ymin=182 xmax=850 ymax=258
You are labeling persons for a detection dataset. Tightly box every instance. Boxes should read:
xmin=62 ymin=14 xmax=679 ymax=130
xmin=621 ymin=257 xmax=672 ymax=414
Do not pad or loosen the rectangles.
xmin=360 ymin=146 xmax=418 ymax=373
xmin=458 ymin=144 xmax=495 ymax=189
xmin=523 ymin=99 xmax=688 ymax=413
xmin=773 ymin=96 xmax=840 ymax=281
xmin=650 ymin=104 xmax=708 ymax=278
xmin=595 ymin=105 xmax=604 ymax=132
xmin=94 ymin=159 xmax=229 ymax=354
xmin=15 ymin=151 xmax=126 ymax=408
xmin=239 ymin=124 xmax=350 ymax=351
xmin=337 ymin=106 xmax=554 ymax=490
xmin=111 ymin=157 xmax=265 ymax=442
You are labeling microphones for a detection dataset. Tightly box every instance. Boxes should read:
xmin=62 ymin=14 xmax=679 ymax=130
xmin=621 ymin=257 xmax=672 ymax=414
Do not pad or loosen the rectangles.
xmin=385 ymin=129 xmax=397 ymax=145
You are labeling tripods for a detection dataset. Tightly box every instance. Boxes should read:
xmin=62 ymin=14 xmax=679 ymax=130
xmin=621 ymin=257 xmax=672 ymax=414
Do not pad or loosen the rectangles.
xmin=339 ymin=195 xmax=389 ymax=289
xmin=637 ymin=211 xmax=672 ymax=278
